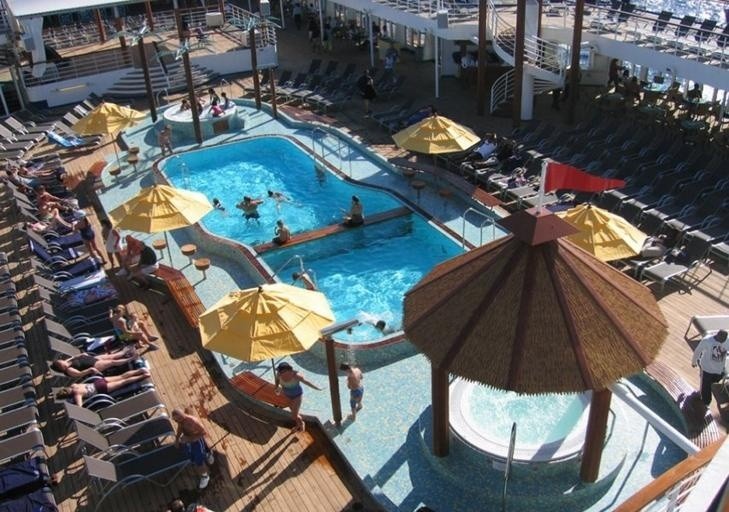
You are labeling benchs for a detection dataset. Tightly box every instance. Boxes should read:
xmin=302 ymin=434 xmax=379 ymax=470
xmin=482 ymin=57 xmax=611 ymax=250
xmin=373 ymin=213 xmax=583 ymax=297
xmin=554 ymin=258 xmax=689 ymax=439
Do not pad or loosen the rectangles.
xmin=117 ymin=249 xmax=207 ymax=328
xmin=227 ymin=371 xmax=291 ymax=408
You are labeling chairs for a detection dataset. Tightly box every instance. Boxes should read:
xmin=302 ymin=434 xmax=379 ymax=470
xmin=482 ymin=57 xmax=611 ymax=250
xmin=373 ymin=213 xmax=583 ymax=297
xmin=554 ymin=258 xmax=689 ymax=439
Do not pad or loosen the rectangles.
xmin=0 ymin=99 xmax=192 ymax=512
xmin=245 ymin=59 xmax=405 ymax=115
xmin=542 ymin=0 xmax=729 ymax=68
xmin=371 ymin=105 xmax=729 ymax=291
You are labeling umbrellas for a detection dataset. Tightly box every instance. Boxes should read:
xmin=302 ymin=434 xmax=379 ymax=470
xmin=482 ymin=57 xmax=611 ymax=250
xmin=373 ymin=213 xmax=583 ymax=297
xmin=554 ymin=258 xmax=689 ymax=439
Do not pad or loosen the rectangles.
xmin=555 ymin=202 xmax=647 ymax=262
xmin=199 ymin=283 xmax=334 ymax=388
xmin=109 ymin=180 xmax=215 ymax=268
xmin=392 ymin=112 xmax=482 ymax=178
xmin=71 ymin=99 xmax=145 ymax=169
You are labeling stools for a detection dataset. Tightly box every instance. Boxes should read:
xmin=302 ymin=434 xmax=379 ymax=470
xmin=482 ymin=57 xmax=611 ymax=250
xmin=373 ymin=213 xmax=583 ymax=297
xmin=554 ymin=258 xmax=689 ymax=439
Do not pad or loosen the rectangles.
xmin=195 ymin=258 xmax=210 ymax=270
xmin=181 ymin=244 xmax=196 ymax=255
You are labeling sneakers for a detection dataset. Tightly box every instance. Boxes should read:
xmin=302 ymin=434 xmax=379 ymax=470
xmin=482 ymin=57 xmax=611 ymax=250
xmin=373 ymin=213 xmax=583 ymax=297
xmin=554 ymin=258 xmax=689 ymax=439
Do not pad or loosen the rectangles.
xmin=199 ymin=475 xmax=210 ymax=488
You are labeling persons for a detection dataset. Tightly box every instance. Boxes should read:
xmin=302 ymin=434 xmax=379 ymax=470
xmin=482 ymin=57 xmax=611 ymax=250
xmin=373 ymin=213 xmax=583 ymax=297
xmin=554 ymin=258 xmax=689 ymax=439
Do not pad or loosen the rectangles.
xmin=51 ymin=304 xmax=159 ymax=406
xmin=172 ymin=408 xmax=215 ymax=489
xmin=280 ymin=0 xmax=401 ymax=83
xmin=465 ymin=132 xmax=532 ymax=186
xmin=159 ymin=125 xmax=174 ymax=155
xmin=340 ymin=361 xmax=364 ymax=421
xmin=647 ymin=233 xmax=688 ymax=265
xmin=344 ymin=195 xmax=365 ymax=225
xmin=275 ymin=362 xmax=322 ymax=433
xmin=7 ymin=160 xmax=158 ymax=290
xmin=170 ymin=497 xmax=198 ymax=512
xmin=364 ymin=311 xmax=394 ymax=335
xmin=212 ymin=190 xmax=291 ymax=245
xmin=181 ymin=87 xmax=230 ymax=118
xmin=401 ymin=105 xmax=434 ymax=127
xmin=357 ymin=68 xmax=376 ymax=119
xmin=183 ymin=17 xmax=214 ymax=42
xmin=292 ymin=271 xmax=315 ymax=290
xmin=691 ymin=328 xmax=729 ymax=404
xmin=552 ymin=58 xmax=702 ymax=111
